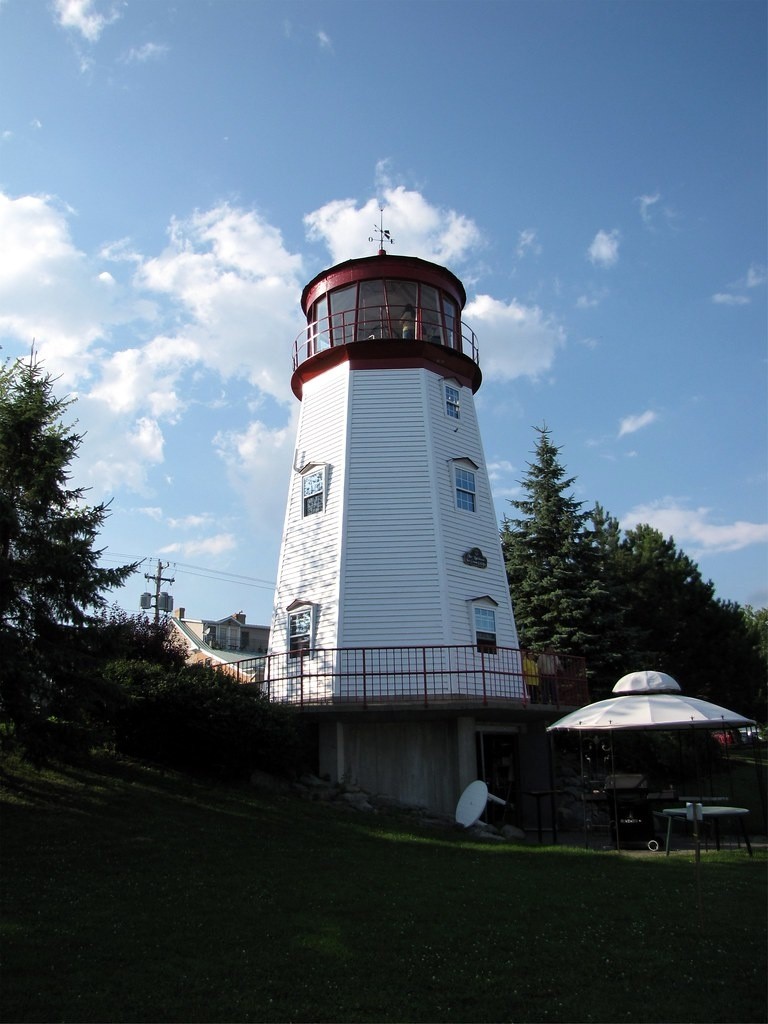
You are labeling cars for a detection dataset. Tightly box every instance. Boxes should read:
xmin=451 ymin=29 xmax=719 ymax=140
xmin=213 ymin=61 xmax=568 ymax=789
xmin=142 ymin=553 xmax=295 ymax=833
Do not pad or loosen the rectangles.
xmin=710 ymin=729 xmax=764 ymax=745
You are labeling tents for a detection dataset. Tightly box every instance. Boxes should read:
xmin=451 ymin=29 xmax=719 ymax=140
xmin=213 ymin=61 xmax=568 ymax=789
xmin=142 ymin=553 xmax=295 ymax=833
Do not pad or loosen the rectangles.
xmin=546 ymin=670 xmax=768 ymax=853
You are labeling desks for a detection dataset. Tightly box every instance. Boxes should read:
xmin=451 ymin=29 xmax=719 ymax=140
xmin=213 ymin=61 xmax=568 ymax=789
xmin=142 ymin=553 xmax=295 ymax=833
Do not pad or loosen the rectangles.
xmin=662 ymin=806 xmax=753 ymax=859
xmin=678 ymin=797 xmax=729 ymax=851
xmin=455 ymin=780 xmax=507 ymax=828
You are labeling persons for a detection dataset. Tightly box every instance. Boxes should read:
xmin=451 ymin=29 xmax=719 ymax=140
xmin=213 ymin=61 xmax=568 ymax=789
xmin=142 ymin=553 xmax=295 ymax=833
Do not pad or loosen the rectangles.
xmin=523 ymin=645 xmax=560 ymax=704
xmin=399 ymin=304 xmax=415 ymax=338
